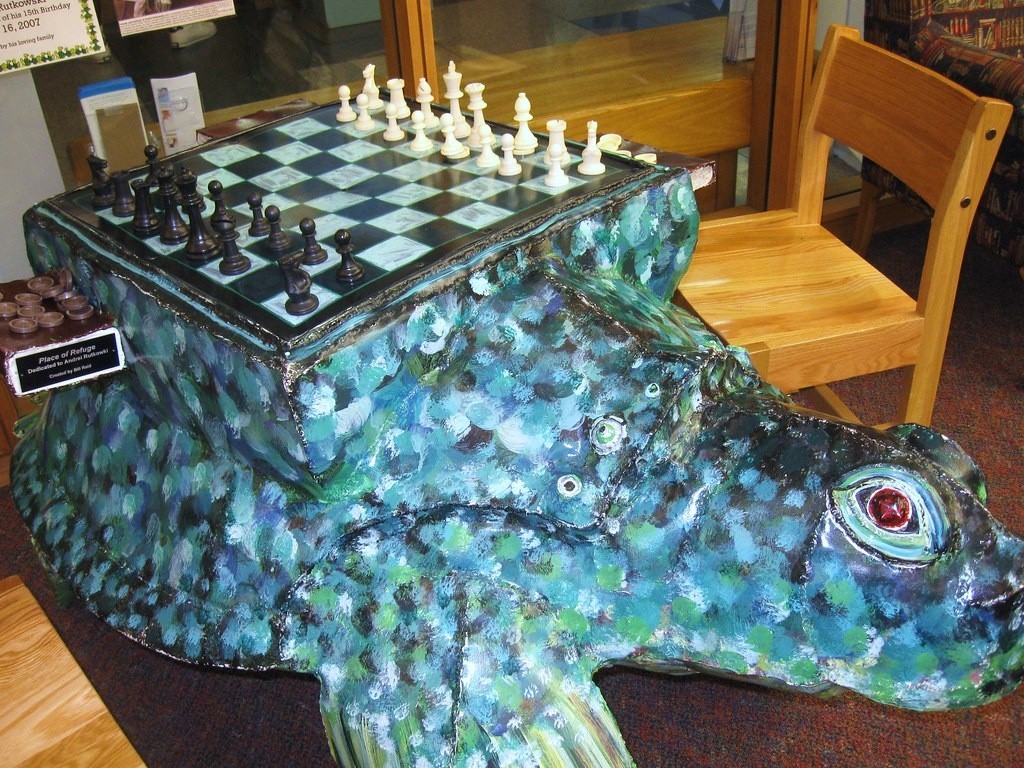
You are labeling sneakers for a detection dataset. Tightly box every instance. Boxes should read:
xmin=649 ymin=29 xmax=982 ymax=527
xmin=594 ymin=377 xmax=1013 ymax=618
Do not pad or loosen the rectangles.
xmin=169 ymin=21 xmax=217 ymax=49
xmin=79 ymin=42 xmax=114 ymax=63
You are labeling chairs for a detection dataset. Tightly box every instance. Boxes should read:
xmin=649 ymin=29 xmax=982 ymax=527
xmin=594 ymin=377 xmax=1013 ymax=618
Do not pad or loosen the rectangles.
xmin=669 ymin=26 xmax=1012 ymax=429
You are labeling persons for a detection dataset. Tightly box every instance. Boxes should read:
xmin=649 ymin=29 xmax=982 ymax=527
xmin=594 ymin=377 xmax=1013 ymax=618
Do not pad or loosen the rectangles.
xmin=60 ymin=0 xmax=218 ymax=64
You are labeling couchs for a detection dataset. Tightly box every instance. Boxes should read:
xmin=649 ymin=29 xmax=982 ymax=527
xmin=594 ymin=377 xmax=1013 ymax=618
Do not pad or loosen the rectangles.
xmin=855 ymin=0 xmax=1024 ymax=311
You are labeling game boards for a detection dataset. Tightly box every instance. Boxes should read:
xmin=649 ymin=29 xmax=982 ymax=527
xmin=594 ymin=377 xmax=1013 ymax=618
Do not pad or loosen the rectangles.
xmin=44 ymin=89 xmax=654 ymax=341
